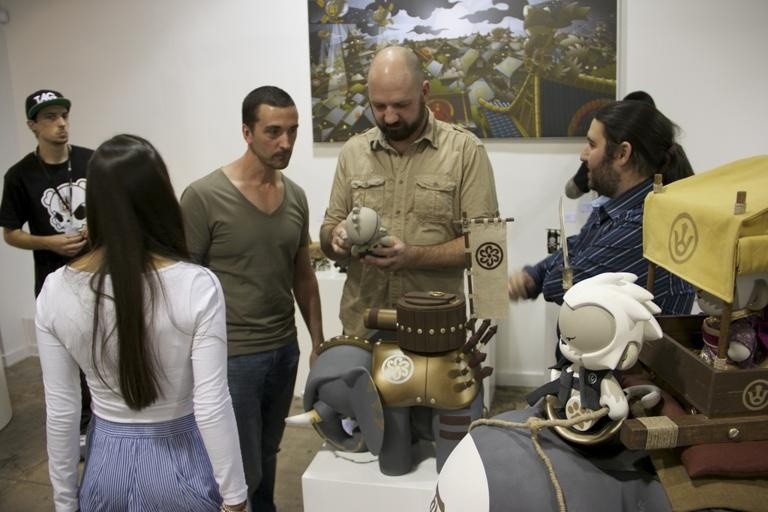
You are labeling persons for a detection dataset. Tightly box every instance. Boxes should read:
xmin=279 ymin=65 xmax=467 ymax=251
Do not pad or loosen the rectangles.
xmin=32 ymin=134 xmax=249 ymax=512
xmin=318 ymin=45 xmax=501 ymax=341
xmin=176 ymin=86 xmax=326 ymax=512
xmin=563 ymin=91 xmax=694 ymax=196
xmin=526 ymin=273 xmax=664 ymax=443
xmin=506 ymin=102 xmax=697 ymax=319
xmin=2 ymin=90 xmax=99 ymax=458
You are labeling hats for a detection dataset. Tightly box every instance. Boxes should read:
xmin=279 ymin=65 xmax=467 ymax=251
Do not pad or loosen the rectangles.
xmin=26 ymin=89 xmax=71 ymax=120
